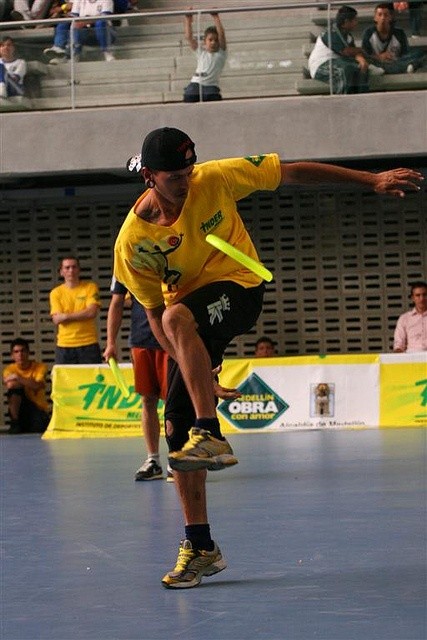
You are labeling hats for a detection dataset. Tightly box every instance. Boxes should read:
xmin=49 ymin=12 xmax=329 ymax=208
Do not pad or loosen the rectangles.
xmin=126 ymin=127 xmax=198 ymax=175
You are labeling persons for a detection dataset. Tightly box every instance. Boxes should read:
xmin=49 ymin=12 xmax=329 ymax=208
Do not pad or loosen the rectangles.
xmin=180 ymin=6 xmax=227 ymax=103
xmin=3 ymin=337 xmax=52 ymax=434
xmin=255 ymin=336 xmax=279 ymax=357
xmin=10 ymin=0 xmax=137 ymax=64
xmin=392 ymin=281 xmax=427 ymax=352
xmin=362 ymin=4 xmax=427 ymax=73
xmin=102 ymin=275 xmax=175 ymax=482
xmin=49 ymin=257 xmax=102 ymax=364
xmin=111 ymin=126 xmax=424 ymax=590
xmin=0 ymin=37 xmax=28 ymax=99
xmin=392 ymin=0 xmax=427 ymax=36
xmin=307 ymin=4 xmax=371 ymax=95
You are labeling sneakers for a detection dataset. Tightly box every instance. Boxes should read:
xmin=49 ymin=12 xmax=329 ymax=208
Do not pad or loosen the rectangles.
xmin=161 ymin=538 xmax=227 ymax=589
xmin=48 ymin=54 xmax=69 ymax=65
xmin=134 ymin=458 xmax=164 ymax=481
xmin=10 ymin=10 xmax=37 ymax=29
xmin=103 ymin=51 xmax=114 ymax=62
xmin=166 ymin=464 xmax=175 ymax=483
xmin=167 ymin=426 xmax=239 ymax=472
xmin=43 ymin=46 xmax=67 ymax=58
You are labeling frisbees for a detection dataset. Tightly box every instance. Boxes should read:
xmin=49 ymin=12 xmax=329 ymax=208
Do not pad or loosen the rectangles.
xmin=108 ymin=357 xmax=130 ymax=399
xmin=206 ymin=233 xmax=273 ymax=283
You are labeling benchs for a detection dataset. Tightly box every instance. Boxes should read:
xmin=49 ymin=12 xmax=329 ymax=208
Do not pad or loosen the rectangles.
xmin=0 ymin=27 xmax=56 ymax=41
xmin=296 ymin=0 xmax=427 ymax=94
xmin=26 ymin=60 xmax=49 ymax=76
xmin=0 ymin=96 xmax=34 ymax=111
